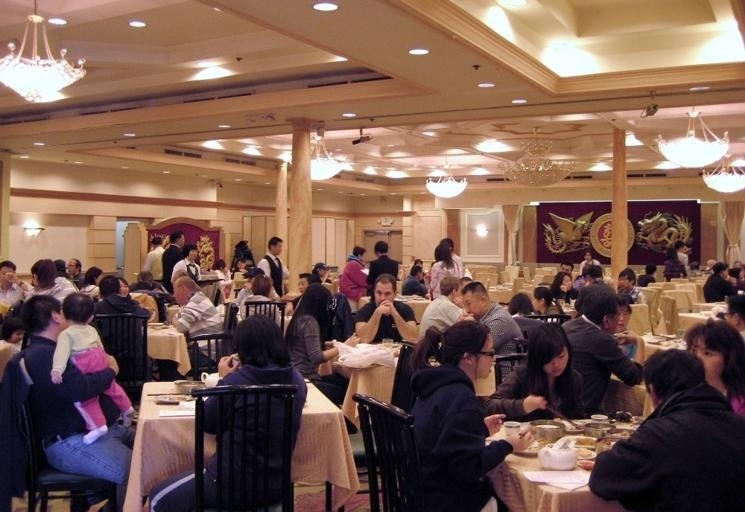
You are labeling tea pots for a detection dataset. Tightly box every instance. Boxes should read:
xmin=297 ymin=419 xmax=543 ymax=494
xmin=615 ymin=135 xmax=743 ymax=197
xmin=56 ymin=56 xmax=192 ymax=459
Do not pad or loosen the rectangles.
xmin=201 ymin=372 xmax=222 ymax=387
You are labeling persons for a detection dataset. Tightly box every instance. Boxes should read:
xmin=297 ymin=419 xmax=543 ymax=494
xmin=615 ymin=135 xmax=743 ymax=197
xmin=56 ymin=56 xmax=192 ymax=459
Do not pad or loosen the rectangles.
xmin=418 ymin=277 xmax=475 ymax=342
xmin=312 ymin=262 xmax=332 ymax=284
xmin=608 ymin=295 xmax=631 ymax=333
xmin=212 ymin=259 xmax=233 ymax=287
xmin=559 ymin=261 xmax=574 ymax=277
xmin=429 ymin=244 xmax=461 ymax=300
xmin=116 ymin=277 xmax=130 ymax=298
xmin=0 ymin=260 xmax=33 ymax=309
xmin=365 ymin=240 xmax=398 ymax=303
xmin=171 ymin=243 xmax=201 ymax=285
xmin=414 ymin=258 xmax=423 ymax=270
xmin=257 ymin=237 xmax=289 ymax=298
xmin=411 ymin=320 xmax=535 ymax=511
xmin=572 ymin=264 xmax=590 ymax=290
xmin=50 ymin=293 xmax=136 ymax=447
xmin=616 ymin=267 xmax=647 ymax=304
xmin=508 ymin=293 xmax=540 ymax=321
xmin=484 ymin=323 xmax=586 ymax=420
xmin=352 ymin=274 xmax=420 ymax=344
xmin=580 ymin=251 xmax=600 ymax=275
xmin=165 ymin=276 xmax=224 ymax=381
xmin=636 ymin=263 xmax=657 ymax=287
xmin=92 ymin=275 xmax=153 ymax=383
xmin=67 ymin=259 xmax=84 ymax=290
xmin=577 ymin=265 xmax=616 ymax=318
xmin=237 ymin=268 xmax=281 ymax=306
xmin=532 ymin=287 xmax=565 ymax=316
xmin=587 ymin=347 xmax=745 ymax=512
xmin=143 ymin=237 xmax=166 ymax=284
xmin=439 ymin=238 xmax=463 ymax=280
xmin=663 ymin=247 xmax=688 ymax=282
xmin=239 ymin=276 xmax=281 ymax=327
xmin=402 ymin=265 xmax=428 ymax=296
xmin=728 ymin=267 xmax=745 ymax=292
xmin=0 ymin=316 xmax=24 ymax=382
xmin=23 ymin=259 xmax=77 ymax=305
xmin=684 ymin=321 xmax=744 ymax=417
xmin=80 ymin=267 xmax=104 ymax=302
xmin=285 ymin=284 xmax=350 ymax=404
xmin=674 ymin=240 xmax=688 ymax=267
xmin=702 ymin=261 xmax=745 ymax=304
xmin=231 ymin=258 xmax=248 ymax=279
xmin=339 ymin=246 xmax=372 ymax=302
xmin=162 ymin=231 xmax=184 ymax=292
xmin=560 ymin=290 xmax=645 ymax=415
xmin=724 ymin=295 xmax=745 ymax=338
xmin=285 ymin=273 xmax=312 ymax=317
xmin=456 ymin=281 xmax=525 ymax=383
xmin=23 ymin=295 xmax=137 ymax=512
xmin=149 ymin=315 xmax=308 ymax=511
xmin=548 ymin=272 xmax=578 ymax=304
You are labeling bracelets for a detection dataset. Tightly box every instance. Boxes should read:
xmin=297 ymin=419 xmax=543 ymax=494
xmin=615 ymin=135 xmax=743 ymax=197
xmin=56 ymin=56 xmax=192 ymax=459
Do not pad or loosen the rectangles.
xmin=17 ymin=279 xmax=23 ymax=287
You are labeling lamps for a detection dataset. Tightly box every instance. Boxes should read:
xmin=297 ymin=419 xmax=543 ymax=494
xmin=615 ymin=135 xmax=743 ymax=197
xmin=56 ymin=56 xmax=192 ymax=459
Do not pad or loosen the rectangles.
xmin=654 ymin=105 xmax=730 ymax=168
xmin=425 ymin=157 xmax=468 ymax=199
xmin=22 ymin=216 xmax=46 ymax=237
xmin=0 ymin=0 xmax=86 ymax=104
xmin=306 ymin=132 xmax=344 ymax=181
xmin=703 ymin=154 xmax=745 ymax=192
xmin=498 ymin=127 xmax=576 ymax=188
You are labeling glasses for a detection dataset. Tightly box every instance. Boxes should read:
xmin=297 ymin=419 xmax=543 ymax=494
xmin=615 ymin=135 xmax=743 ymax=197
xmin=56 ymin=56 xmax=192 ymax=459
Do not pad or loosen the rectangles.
xmin=467 ymin=349 xmax=496 ymax=357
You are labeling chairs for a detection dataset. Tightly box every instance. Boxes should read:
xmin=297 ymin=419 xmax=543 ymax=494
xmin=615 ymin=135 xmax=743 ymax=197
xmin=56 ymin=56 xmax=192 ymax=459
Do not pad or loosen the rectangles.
xmin=628 ymin=303 xmax=650 ymax=336
xmin=672 ymin=273 xmax=709 ymax=285
xmin=663 ymin=290 xmax=693 ymax=314
xmin=193 ymin=384 xmax=297 ymax=512
xmin=72 ymin=279 xmax=78 ymax=284
xmin=514 ymin=277 xmax=532 ymax=298
xmin=525 ymin=314 xmax=571 ymax=325
xmin=637 ymin=288 xmax=657 ymax=322
xmin=352 ymin=393 xmax=414 ymax=512
xmin=485 ymin=278 xmax=490 ymax=291
xmin=676 ymin=283 xmax=697 ymax=303
xmin=245 ymin=301 xmax=286 ymax=334
xmin=650 ymin=282 xmax=676 ymax=292
xmin=475 ymin=263 xmax=519 ymax=282
xmin=138 ymin=290 xmax=165 ymax=322
xmin=233 ymin=288 xmax=240 ymax=298
xmin=96 ymin=313 xmax=149 ymax=404
xmin=659 ymin=294 xmax=679 ymax=334
xmin=514 ymin=337 xmax=532 ymax=365
xmin=532 ymin=267 xmax=557 ymax=282
xmin=331 ymin=278 xmax=340 ymax=295
xmin=189 ymin=302 xmax=239 ymax=381
xmin=647 ymin=286 xmax=663 ymax=306
xmin=322 ymin=281 xmax=330 ymax=294
xmin=7 ymin=346 xmax=119 ymax=512
xmin=495 ymin=353 xmax=529 ymax=391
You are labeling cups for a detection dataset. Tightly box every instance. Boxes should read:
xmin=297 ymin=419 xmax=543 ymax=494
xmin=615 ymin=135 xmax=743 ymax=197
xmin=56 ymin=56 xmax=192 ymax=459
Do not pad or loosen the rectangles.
xmin=592 ymin=415 xmax=608 ymax=422
xmin=503 ymin=422 xmax=519 ymax=434
xmin=230 ymin=353 xmax=241 ymax=368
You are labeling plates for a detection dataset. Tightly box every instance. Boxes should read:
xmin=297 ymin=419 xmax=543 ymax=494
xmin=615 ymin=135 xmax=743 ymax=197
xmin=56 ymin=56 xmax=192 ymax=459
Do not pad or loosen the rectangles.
xmin=543 ymin=443 xmax=598 ymax=460
xmin=562 ymin=436 xmax=597 ymax=448
xmin=513 ymin=442 xmax=544 ymax=454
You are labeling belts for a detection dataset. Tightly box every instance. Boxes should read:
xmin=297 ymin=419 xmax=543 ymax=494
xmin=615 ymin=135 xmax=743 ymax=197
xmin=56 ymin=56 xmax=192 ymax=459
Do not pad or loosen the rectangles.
xmin=41 ymin=430 xmax=84 ymax=448
